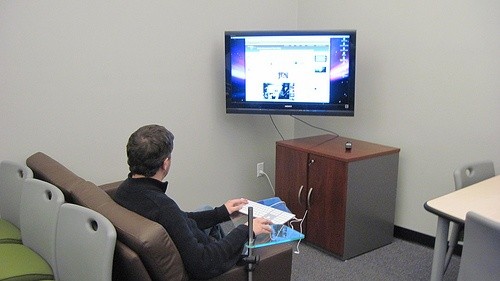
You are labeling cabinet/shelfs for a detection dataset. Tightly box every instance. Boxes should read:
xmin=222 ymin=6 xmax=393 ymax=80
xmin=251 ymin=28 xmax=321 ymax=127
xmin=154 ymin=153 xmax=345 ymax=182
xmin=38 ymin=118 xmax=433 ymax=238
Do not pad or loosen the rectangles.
xmin=274 ymin=133 xmax=401 ymax=262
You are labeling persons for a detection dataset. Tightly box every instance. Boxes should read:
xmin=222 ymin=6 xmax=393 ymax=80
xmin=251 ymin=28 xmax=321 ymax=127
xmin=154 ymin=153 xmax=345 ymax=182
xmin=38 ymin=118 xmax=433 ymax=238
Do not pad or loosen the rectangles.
xmin=114 ymin=125 xmax=273 ymax=281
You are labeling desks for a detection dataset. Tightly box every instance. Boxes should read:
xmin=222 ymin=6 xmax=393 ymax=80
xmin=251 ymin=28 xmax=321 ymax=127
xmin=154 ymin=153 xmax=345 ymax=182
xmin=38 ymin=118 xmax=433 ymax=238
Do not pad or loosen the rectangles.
xmin=423 ymin=173 xmax=500 ymax=281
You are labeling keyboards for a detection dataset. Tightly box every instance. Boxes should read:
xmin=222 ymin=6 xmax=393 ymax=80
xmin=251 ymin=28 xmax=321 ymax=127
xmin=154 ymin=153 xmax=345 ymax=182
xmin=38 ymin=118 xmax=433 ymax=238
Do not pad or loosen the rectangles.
xmin=234 ymin=196 xmax=296 ymax=226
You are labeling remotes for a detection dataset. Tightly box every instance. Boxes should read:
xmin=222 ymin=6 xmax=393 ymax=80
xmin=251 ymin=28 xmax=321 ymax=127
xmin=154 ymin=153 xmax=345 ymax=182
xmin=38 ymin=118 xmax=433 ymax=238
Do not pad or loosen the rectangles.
xmin=345 ymin=140 xmax=352 ymax=150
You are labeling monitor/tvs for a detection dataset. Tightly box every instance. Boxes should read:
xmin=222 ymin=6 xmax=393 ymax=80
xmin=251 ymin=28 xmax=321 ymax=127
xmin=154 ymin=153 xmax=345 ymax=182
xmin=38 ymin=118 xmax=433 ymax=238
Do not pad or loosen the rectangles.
xmin=225 ymin=31 xmax=355 ymax=116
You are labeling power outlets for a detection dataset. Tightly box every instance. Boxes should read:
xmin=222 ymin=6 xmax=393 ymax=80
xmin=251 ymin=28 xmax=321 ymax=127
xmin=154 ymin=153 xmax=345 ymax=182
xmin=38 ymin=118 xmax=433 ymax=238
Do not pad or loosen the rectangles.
xmin=256 ymin=161 xmax=265 ymax=178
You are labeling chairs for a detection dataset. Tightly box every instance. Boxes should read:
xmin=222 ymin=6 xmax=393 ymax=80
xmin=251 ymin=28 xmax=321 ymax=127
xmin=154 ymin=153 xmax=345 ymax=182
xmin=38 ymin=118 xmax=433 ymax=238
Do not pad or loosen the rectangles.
xmin=454 ymin=211 xmax=500 ymax=281
xmin=444 ymin=159 xmax=496 ymax=272
xmin=0 ymin=151 xmax=294 ymax=281
